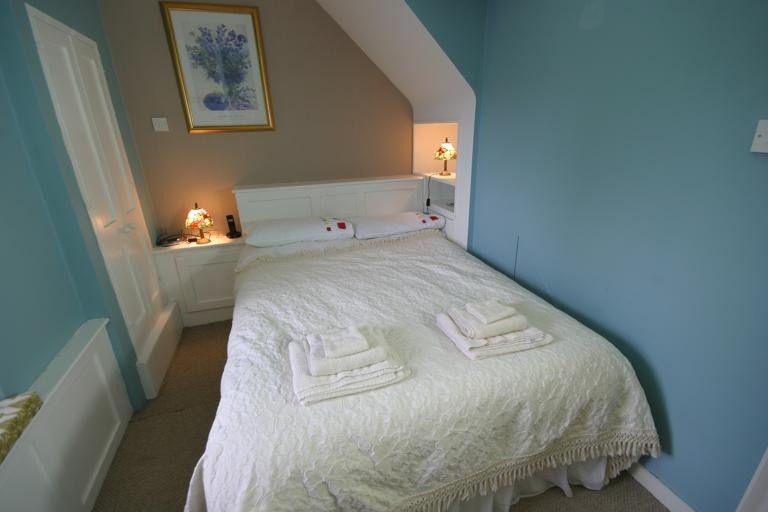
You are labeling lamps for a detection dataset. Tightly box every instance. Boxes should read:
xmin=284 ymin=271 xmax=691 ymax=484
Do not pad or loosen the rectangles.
xmin=184 ymin=202 xmax=215 ymax=245
xmin=433 ymin=137 xmax=458 ymax=176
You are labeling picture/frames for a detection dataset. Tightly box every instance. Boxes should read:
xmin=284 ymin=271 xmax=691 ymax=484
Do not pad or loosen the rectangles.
xmin=157 ymin=0 xmax=275 ymax=135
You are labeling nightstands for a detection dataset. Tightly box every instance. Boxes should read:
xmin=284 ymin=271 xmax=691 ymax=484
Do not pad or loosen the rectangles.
xmin=154 ymin=229 xmax=244 ymax=329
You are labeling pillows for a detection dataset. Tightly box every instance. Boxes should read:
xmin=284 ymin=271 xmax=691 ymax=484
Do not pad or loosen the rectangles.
xmin=243 ymin=212 xmax=446 ymax=249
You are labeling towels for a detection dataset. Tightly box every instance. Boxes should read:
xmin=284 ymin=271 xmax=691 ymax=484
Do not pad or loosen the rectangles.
xmin=287 ymin=298 xmax=553 ymax=406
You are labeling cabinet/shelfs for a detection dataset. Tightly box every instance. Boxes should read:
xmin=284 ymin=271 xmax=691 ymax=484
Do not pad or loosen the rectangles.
xmin=412 ymin=173 xmax=456 ymax=221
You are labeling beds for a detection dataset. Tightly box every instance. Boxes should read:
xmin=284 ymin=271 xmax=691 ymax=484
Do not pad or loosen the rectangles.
xmin=198 ymin=172 xmax=638 ymax=511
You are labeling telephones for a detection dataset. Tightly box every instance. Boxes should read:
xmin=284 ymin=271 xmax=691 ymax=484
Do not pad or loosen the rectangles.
xmin=226 ymin=215 xmax=241 ymax=238
